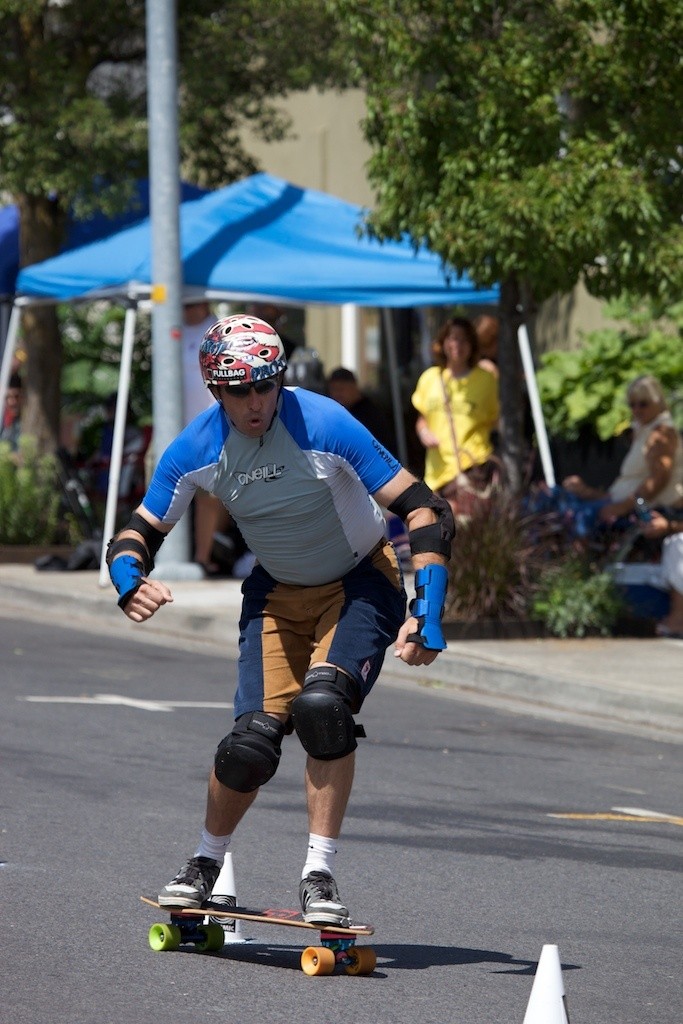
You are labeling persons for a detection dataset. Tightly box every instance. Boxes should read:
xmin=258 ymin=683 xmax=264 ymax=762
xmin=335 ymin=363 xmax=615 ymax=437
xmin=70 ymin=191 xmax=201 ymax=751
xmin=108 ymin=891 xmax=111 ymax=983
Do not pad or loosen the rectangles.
xmin=413 ymin=317 xmax=497 ymax=515
xmin=559 ymin=375 xmax=683 ymax=636
xmin=326 ymin=366 xmax=394 ymax=450
xmin=279 ymin=335 xmax=326 ymax=398
xmin=184 ymin=302 xmax=224 ymax=576
xmin=84 ymin=394 xmax=143 ymax=498
xmin=2 ymin=375 xmax=24 ymax=452
xmin=108 ymin=315 xmax=458 ymax=924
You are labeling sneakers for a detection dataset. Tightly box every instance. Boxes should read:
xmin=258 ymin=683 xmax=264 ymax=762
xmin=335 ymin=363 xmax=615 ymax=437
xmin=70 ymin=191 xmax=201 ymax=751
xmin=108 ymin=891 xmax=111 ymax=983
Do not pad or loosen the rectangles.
xmin=299 ymin=871 xmax=351 ymax=925
xmin=158 ymin=856 xmax=221 ymax=909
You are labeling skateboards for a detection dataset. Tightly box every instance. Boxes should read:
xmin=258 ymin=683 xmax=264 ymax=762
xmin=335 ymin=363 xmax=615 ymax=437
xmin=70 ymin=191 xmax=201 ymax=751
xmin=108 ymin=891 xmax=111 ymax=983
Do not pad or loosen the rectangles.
xmin=141 ymin=894 xmax=377 ymax=977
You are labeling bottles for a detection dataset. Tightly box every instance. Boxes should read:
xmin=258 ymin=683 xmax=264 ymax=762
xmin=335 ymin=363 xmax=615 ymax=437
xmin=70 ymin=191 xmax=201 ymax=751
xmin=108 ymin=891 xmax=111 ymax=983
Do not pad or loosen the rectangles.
xmin=635 ymin=494 xmax=651 ymax=521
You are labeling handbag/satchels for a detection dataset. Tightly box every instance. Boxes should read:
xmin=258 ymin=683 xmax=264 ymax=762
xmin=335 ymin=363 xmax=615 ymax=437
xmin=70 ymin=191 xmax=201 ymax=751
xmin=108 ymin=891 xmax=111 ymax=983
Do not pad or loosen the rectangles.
xmin=452 ymin=473 xmax=492 ymax=521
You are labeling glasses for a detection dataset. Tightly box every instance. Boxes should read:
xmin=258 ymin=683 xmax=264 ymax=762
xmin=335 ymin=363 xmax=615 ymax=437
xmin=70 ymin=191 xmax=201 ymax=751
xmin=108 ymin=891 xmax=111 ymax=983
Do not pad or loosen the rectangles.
xmin=628 ymin=397 xmax=651 ymax=409
xmin=226 ymin=378 xmax=278 ymax=398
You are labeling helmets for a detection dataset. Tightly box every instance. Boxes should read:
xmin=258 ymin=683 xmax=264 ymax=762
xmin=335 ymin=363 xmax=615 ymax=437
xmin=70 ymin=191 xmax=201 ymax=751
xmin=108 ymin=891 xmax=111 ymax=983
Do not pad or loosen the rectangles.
xmin=199 ymin=314 xmax=287 ymax=385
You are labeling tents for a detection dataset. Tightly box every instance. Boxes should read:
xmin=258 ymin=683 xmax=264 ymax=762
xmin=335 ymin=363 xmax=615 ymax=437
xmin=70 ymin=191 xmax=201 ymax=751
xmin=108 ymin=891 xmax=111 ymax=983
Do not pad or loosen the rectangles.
xmin=0 ymin=173 xmax=210 ymax=313
xmin=1 ymin=173 xmax=555 ymax=590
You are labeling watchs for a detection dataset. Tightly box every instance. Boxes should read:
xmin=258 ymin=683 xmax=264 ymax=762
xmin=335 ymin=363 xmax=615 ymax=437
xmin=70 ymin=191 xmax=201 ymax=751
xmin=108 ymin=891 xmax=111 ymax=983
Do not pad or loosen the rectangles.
xmin=635 ymin=493 xmax=645 ymax=505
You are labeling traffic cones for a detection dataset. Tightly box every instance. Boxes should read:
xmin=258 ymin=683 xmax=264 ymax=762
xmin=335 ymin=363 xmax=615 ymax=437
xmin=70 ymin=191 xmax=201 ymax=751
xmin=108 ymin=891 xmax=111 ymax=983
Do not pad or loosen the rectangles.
xmin=520 ymin=945 xmax=572 ymax=1023
xmin=201 ymin=851 xmax=247 ymax=944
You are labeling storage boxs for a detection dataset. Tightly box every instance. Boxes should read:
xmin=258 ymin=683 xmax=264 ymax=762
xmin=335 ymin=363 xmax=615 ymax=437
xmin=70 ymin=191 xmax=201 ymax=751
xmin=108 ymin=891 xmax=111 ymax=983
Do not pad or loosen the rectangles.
xmin=600 ymin=564 xmax=675 ymax=620
